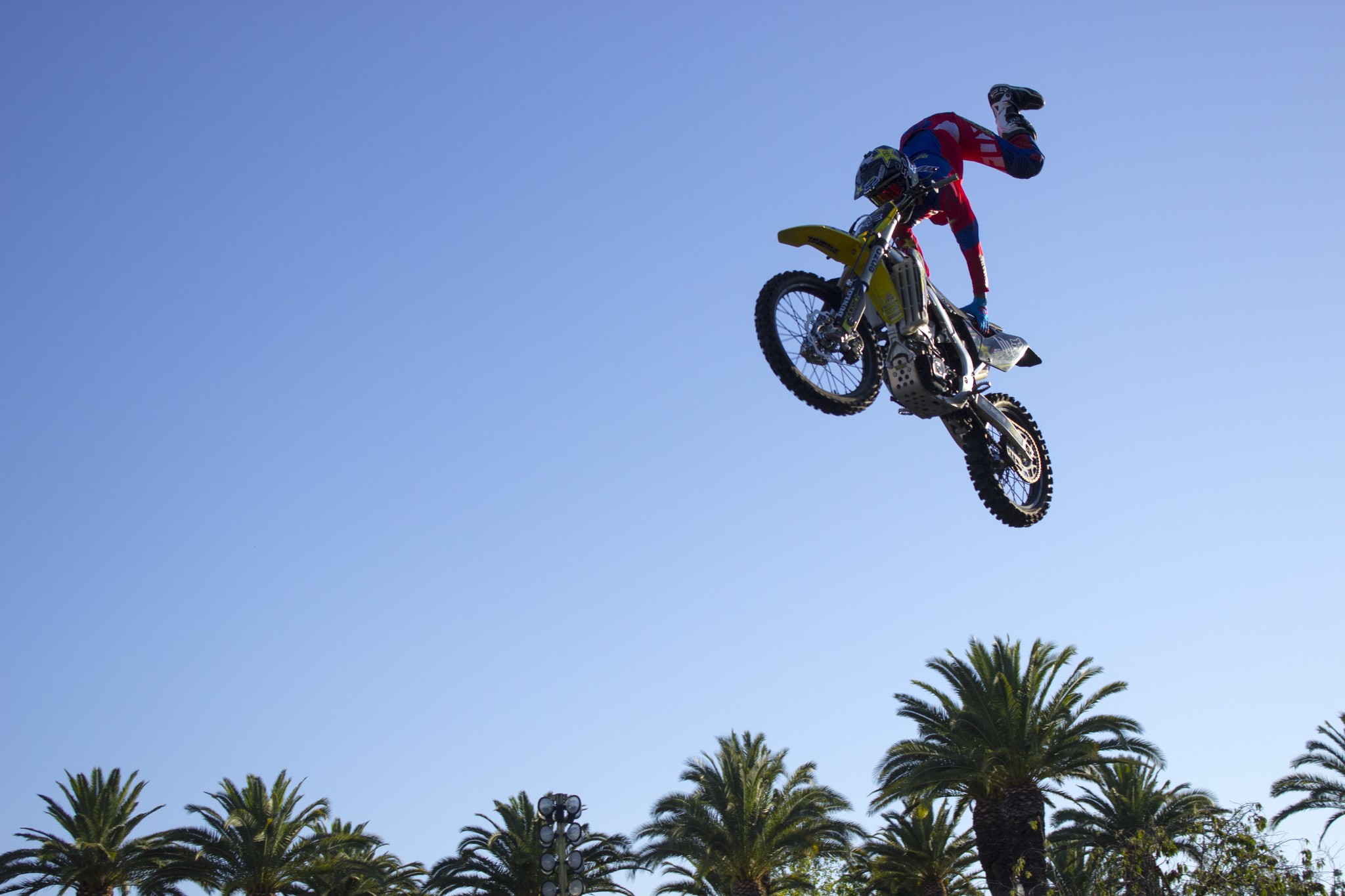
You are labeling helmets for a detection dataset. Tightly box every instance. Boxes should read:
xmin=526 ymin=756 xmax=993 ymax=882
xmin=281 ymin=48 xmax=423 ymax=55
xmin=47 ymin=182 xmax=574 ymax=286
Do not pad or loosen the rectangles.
xmin=853 ymin=145 xmax=917 ymax=223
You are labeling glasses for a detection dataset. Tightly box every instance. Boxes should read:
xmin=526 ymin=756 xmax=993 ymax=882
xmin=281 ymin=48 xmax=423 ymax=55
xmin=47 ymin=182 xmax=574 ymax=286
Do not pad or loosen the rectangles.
xmin=866 ymin=173 xmax=909 ymax=207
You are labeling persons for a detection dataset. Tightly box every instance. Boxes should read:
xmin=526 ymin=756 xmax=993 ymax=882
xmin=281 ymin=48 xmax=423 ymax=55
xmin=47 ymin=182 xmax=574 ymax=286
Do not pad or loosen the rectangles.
xmin=854 ymin=82 xmax=1048 ymax=337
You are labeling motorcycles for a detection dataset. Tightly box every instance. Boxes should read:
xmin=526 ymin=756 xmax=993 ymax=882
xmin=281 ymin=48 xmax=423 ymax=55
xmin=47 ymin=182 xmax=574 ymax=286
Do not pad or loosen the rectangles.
xmin=753 ymin=173 xmax=1054 ymax=529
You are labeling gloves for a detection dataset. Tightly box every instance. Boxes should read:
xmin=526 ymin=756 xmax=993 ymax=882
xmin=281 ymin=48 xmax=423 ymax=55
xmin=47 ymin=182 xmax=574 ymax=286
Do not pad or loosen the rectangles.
xmin=959 ymin=297 xmax=990 ymax=334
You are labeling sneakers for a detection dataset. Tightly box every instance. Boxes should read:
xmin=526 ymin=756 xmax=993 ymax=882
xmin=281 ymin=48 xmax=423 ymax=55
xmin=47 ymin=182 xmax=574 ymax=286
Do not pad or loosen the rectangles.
xmin=989 ymin=84 xmax=1046 ymax=111
